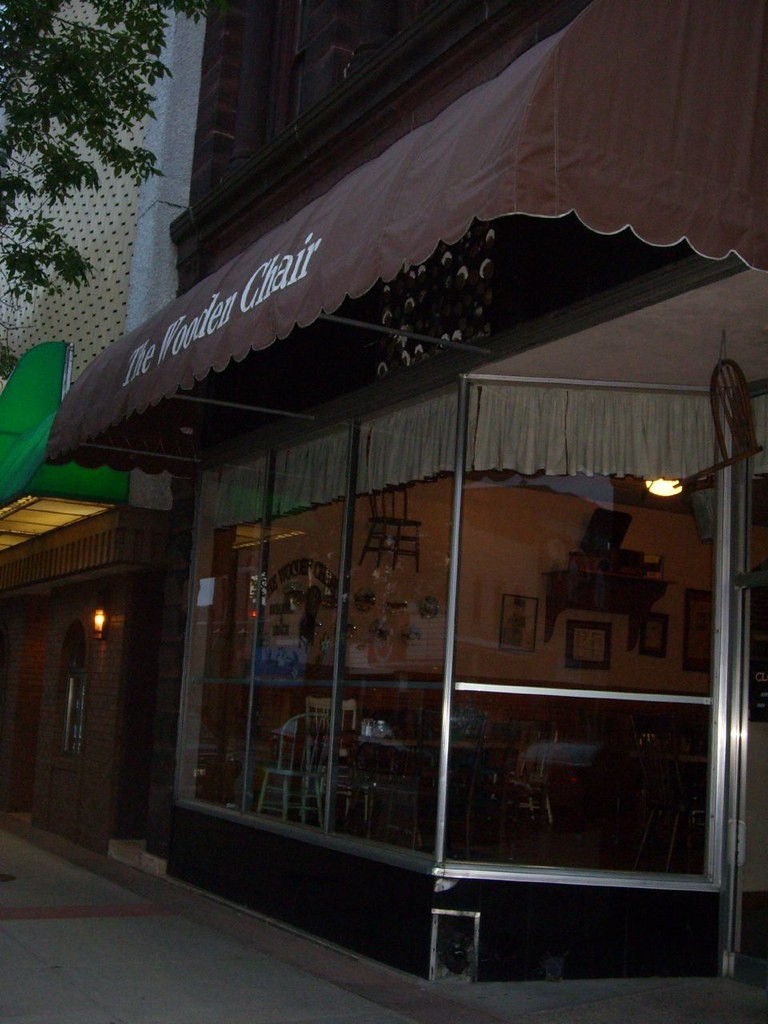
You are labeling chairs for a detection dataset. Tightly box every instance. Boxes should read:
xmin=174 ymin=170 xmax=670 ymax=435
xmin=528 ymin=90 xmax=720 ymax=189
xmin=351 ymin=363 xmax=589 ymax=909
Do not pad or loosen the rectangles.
xmin=358 ymin=483 xmax=422 ymax=574
xmin=252 ymin=689 xmax=563 ymax=864
xmin=627 ymin=708 xmax=707 ymax=879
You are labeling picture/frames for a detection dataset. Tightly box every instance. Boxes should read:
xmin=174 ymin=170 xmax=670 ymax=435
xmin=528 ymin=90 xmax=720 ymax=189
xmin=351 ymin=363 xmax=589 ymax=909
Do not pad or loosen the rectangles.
xmin=638 ymin=610 xmax=670 ymax=659
xmin=565 ymin=619 xmax=612 ymax=671
xmin=683 ymin=587 xmax=714 ymax=677
xmin=498 ymin=593 xmax=539 ymax=654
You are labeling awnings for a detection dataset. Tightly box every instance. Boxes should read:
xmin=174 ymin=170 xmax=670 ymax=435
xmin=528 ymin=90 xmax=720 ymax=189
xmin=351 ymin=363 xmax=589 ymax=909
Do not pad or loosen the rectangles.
xmin=0 ymin=411 xmax=130 ymax=554
xmin=42 ymin=0 xmax=767 ymax=484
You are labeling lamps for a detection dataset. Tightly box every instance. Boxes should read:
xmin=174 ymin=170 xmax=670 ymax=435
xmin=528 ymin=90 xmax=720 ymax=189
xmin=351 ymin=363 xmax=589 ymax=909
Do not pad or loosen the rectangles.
xmin=691 ymin=485 xmax=719 ymax=545
xmin=93 ymin=605 xmax=105 ymax=641
xmin=178 ymin=400 xmax=202 ymax=434
xmin=645 ymin=476 xmax=686 ymax=497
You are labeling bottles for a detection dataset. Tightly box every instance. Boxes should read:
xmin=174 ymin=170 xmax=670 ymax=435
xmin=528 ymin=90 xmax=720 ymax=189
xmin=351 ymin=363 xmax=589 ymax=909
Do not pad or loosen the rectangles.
xmin=638 ymin=730 xmax=659 ymax=755
xmin=361 ymin=706 xmax=397 ymax=739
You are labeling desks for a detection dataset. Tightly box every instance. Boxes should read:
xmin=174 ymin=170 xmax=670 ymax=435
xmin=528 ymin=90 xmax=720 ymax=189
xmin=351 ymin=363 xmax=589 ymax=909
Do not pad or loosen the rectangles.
xmin=350 ymin=736 xmax=482 ymax=850
xmin=629 ymin=750 xmax=711 ymax=874
